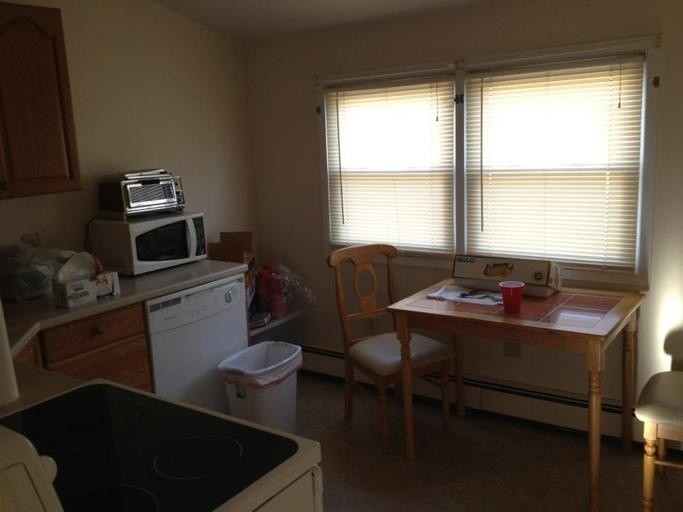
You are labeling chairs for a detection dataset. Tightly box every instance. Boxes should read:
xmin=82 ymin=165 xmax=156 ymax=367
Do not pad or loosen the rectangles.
xmin=325 ymin=242 xmax=458 ymax=461
xmin=632 ymin=370 xmax=683 ymax=512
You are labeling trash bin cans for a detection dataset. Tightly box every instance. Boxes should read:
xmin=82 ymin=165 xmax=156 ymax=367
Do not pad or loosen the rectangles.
xmin=217 ymin=341 xmax=303 ymax=433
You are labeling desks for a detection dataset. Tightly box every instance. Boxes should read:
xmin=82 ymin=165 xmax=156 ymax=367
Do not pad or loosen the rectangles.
xmin=248 ymin=305 xmax=305 ymax=345
xmin=384 ymin=277 xmax=648 ymax=511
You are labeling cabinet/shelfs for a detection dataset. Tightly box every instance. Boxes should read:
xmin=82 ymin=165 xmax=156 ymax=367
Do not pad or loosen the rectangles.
xmin=40 ymin=304 xmax=155 ymax=393
xmin=14 ymin=335 xmax=44 ymax=368
xmin=0 ymin=0 xmax=86 ymax=202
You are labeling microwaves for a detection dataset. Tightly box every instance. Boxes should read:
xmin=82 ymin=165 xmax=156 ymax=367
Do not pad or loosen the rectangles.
xmin=86 ymin=210 xmax=207 ymax=276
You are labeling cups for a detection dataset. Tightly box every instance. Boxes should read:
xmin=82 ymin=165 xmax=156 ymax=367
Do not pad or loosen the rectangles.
xmin=499 ymin=280 xmax=525 ymax=315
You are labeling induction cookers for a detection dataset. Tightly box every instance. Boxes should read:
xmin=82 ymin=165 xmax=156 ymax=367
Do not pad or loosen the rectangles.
xmin=0 ymin=384 xmax=300 ymax=512
